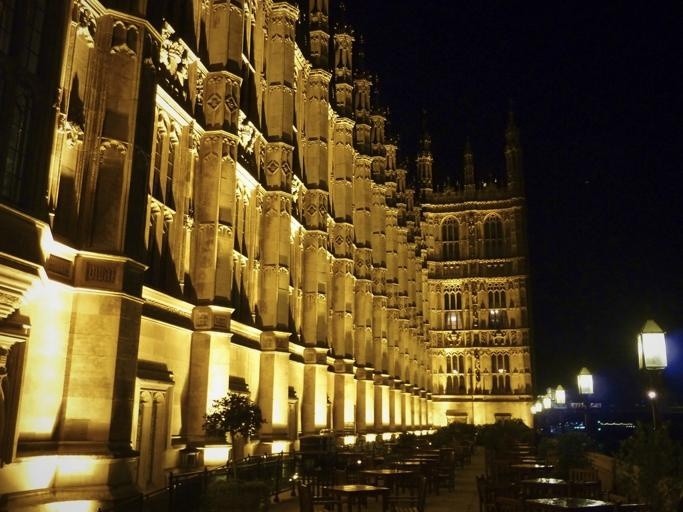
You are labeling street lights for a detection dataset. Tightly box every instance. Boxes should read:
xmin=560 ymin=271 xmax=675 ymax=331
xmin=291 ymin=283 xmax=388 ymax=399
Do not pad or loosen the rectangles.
xmin=576 ymin=366 xmax=599 ymax=446
xmin=636 ymin=317 xmax=668 ymax=429
xmin=530 ymin=384 xmax=568 ymax=434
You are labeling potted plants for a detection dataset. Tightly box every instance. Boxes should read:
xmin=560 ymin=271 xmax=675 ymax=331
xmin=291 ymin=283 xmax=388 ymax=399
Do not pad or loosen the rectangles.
xmin=203 ymin=393 xmax=262 ymax=460
